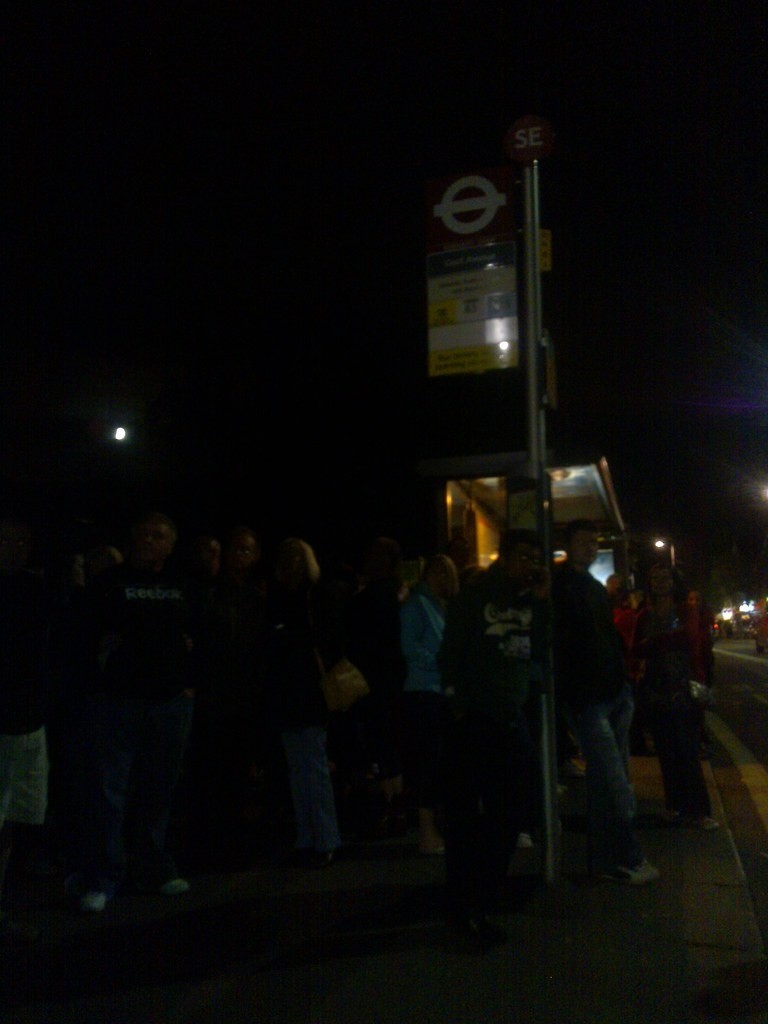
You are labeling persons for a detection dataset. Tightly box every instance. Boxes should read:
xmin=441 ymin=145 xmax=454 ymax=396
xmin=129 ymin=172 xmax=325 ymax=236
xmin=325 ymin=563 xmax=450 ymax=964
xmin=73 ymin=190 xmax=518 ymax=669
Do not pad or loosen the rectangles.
xmin=0 ymin=513 xmax=718 ymax=946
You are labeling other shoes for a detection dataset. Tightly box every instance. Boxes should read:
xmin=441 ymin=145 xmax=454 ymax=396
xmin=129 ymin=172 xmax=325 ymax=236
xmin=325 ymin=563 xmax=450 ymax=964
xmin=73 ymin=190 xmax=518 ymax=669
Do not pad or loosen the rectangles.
xmin=81 ymin=890 xmax=105 ymax=914
xmin=602 ymin=860 xmax=658 ymax=886
xmin=690 ymin=813 xmax=720 ymax=831
xmin=419 ymin=837 xmax=447 ymax=854
xmin=281 ymin=846 xmax=334 ymax=866
xmin=159 ymin=877 xmax=190 ymax=895
xmin=2 ymin=911 xmax=40 ymax=944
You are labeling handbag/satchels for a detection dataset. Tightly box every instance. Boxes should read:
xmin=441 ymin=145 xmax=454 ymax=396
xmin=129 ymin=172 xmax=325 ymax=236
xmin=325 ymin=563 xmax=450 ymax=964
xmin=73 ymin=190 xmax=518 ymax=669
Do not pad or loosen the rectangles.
xmin=322 ymin=655 xmax=370 ymax=711
